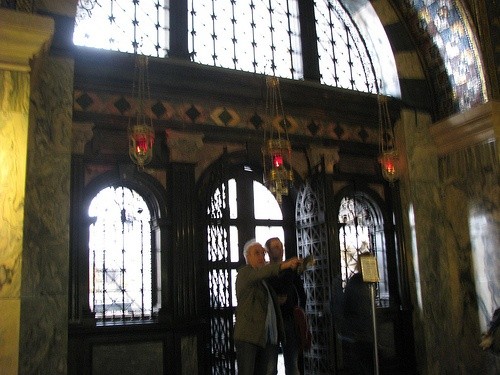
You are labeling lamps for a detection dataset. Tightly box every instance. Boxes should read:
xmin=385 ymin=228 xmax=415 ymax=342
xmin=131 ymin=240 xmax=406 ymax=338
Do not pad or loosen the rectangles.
xmin=264 ymin=76 xmax=297 ymax=207
xmin=374 ymin=93 xmax=400 ymax=184
xmin=126 ymin=53 xmax=158 ymax=177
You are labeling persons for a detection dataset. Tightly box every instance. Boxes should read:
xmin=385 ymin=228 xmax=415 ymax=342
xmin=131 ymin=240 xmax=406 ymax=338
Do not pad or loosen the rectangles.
xmin=235 ymin=237 xmax=308 ymax=375
